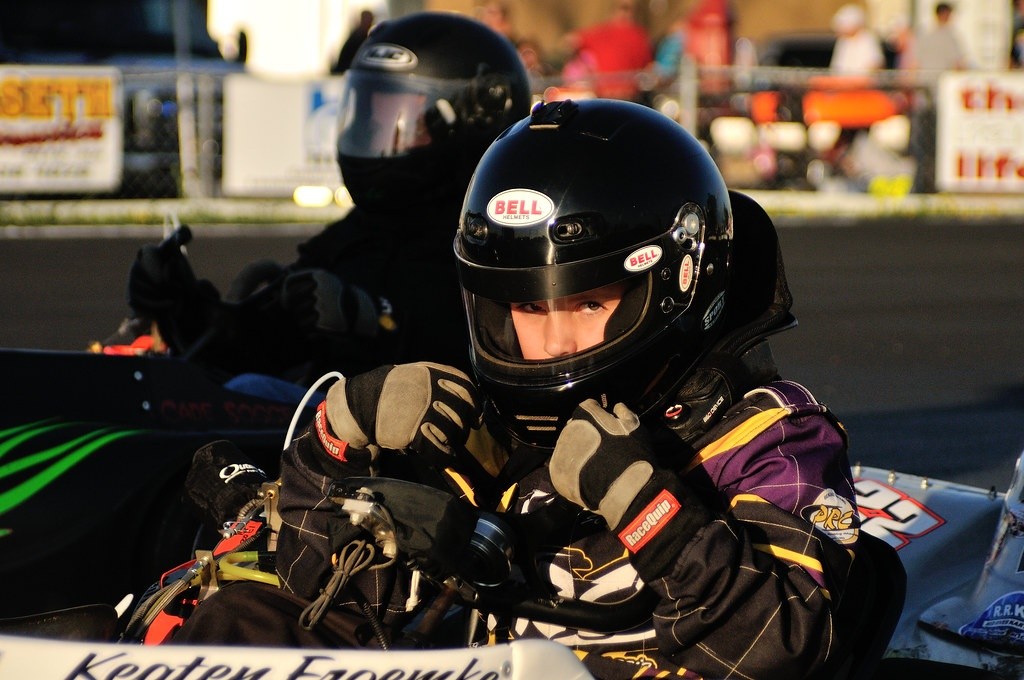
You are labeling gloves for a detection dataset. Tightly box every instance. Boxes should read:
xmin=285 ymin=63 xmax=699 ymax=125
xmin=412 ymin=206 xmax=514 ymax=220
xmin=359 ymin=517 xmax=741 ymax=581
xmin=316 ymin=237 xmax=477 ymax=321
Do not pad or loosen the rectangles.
xmin=127 ymin=242 xmax=219 ymax=355
xmin=281 ymin=267 xmax=378 ymax=342
xmin=547 ymin=393 xmax=708 ymax=585
xmin=325 ymin=361 xmax=482 ymax=474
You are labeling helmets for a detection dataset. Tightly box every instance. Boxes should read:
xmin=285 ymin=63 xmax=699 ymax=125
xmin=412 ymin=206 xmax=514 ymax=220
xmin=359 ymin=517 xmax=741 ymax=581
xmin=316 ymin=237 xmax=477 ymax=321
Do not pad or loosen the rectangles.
xmin=336 ymin=12 xmax=532 ymax=214
xmin=452 ymin=99 xmax=733 ymax=447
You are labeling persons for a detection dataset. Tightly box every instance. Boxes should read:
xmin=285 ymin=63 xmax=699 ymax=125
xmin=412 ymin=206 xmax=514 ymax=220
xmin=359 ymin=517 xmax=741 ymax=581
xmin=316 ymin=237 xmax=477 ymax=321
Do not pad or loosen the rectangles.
xmin=126 ymin=101 xmax=905 ymax=680
xmin=128 ymin=13 xmax=529 ymax=427
xmin=563 ymin=1 xmax=985 ymax=175
